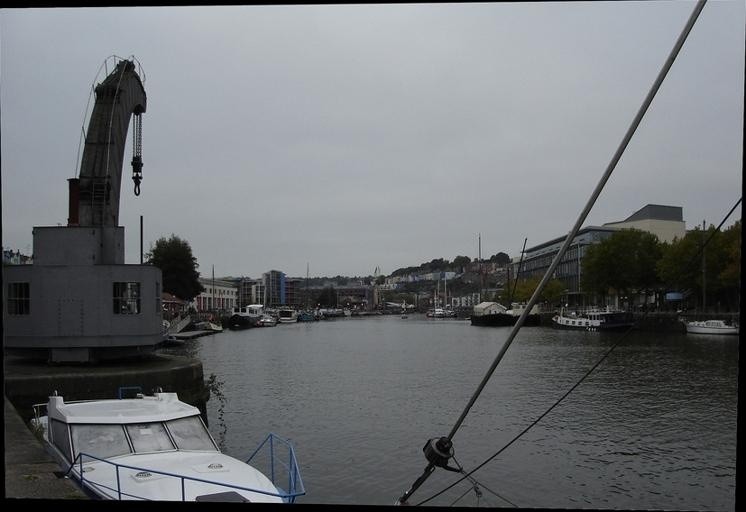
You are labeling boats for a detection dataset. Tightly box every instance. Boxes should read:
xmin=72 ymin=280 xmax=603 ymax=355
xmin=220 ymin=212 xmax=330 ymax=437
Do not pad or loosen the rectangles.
xmin=681 ymin=317 xmax=738 ymax=336
xmin=549 ymin=306 xmax=606 ymax=330
xmin=195 ymin=265 xmax=345 ymax=334
xmin=24 ymin=386 xmax=310 ymax=503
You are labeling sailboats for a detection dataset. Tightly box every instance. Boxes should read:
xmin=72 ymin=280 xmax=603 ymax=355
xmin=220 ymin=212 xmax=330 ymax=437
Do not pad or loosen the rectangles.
xmin=426 ymin=275 xmax=457 ymax=319
xmin=401 ymin=300 xmax=407 ymax=319
